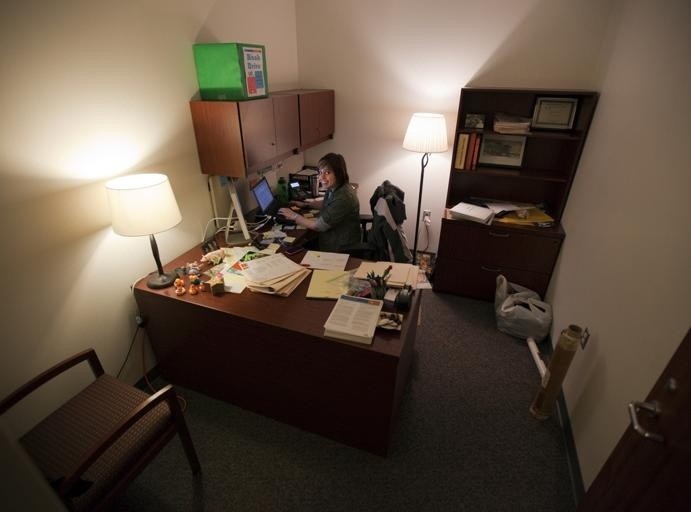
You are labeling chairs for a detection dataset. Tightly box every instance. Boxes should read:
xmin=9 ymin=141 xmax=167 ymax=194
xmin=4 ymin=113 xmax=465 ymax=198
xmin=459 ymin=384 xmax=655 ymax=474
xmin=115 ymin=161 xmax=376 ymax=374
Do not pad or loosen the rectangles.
xmin=352 ymin=180 xmax=408 ymax=257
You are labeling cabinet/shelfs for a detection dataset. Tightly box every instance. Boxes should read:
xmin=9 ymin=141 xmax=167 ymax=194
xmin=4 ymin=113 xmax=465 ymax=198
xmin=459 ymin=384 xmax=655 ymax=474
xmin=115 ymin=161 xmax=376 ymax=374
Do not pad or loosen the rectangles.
xmin=431 ymin=87 xmax=604 ymax=215
xmin=189 ymin=90 xmax=302 ymax=179
xmin=272 ymin=87 xmax=336 ymax=150
xmin=431 ymin=203 xmax=565 ymax=303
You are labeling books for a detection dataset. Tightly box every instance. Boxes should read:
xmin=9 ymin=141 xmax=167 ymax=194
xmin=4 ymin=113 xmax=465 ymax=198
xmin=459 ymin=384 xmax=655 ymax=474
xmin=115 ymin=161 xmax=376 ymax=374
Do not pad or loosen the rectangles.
xmin=450 ymin=199 xmax=556 ymax=230
xmin=216 ymin=247 xmax=434 ymax=346
xmin=454 ymin=110 xmax=532 ymax=172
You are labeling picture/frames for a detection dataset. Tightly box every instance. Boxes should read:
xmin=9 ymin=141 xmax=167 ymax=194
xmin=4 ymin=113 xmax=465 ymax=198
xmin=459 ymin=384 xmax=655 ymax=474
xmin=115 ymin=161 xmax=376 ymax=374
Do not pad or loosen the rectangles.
xmin=478 ymin=129 xmax=530 ymax=171
xmin=530 ymin=90 xmax=585 ymax=134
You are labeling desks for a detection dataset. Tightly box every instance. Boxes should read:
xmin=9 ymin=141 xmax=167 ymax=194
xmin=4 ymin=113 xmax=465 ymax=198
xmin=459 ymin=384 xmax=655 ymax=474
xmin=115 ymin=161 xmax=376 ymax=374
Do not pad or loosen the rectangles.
xmin=132 ymin=239 xmax=422 ymax=458
xmin=207 ymin=176 xmax=358 ymax=255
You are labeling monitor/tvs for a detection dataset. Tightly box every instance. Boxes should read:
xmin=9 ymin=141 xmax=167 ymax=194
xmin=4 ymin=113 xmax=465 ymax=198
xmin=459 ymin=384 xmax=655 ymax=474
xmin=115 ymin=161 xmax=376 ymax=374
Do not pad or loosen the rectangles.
xmin=225 ymin=182 xmax=250 ymax=240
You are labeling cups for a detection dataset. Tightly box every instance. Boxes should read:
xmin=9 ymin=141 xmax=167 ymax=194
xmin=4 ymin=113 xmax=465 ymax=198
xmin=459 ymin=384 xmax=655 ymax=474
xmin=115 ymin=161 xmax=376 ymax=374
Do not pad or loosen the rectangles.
xmin=369 ymin=278 xmax=385 ymax=299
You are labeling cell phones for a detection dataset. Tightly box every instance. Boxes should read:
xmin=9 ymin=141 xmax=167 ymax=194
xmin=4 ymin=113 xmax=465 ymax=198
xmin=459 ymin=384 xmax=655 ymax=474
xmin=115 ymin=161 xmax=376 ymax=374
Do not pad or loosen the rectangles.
xmin=286 ymin=246 xmax=305 ymax=255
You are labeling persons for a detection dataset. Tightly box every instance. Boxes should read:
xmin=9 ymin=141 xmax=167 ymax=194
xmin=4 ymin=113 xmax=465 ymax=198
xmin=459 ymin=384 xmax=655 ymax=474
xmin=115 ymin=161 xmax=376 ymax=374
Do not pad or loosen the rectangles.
xmin=275 ymin=153 xmax=364 ymax=253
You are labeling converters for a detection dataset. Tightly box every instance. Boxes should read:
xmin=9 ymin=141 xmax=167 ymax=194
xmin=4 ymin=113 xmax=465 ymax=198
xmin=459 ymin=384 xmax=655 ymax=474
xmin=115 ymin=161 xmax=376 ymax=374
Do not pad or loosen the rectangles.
xmin=424 ymin=216 xmax=431 ymax=226
xmin=235 ymin=220 xmax=245 ymax=232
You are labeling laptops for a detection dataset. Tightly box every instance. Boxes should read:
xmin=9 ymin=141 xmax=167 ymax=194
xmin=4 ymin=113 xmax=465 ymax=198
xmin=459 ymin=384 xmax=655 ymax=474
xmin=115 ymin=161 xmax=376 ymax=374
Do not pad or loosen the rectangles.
xmin=250 ymin=176 xmax=302 ymax=225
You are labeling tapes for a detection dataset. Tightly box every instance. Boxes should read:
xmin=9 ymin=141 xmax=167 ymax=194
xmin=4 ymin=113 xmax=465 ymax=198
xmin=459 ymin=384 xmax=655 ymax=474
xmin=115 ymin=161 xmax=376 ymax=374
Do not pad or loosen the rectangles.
xmin=403 ymin=282 xmax=412 ymax=289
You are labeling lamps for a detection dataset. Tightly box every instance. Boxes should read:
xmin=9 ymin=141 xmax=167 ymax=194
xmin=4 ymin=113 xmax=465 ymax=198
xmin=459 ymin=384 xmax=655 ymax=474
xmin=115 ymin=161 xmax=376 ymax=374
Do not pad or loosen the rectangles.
xmin=106 ymin=173 xmax=184 ymax=290
xmin=402 ymin=112 xmax=449 ymax=264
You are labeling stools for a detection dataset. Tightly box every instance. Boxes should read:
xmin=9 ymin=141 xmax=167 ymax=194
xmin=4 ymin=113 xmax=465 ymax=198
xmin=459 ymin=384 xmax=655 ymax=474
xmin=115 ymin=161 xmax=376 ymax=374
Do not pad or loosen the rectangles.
xmin=0 ymin=348 xmax=201 ymax=511
xmin=355 ymin=212 xmax=375 ymax=243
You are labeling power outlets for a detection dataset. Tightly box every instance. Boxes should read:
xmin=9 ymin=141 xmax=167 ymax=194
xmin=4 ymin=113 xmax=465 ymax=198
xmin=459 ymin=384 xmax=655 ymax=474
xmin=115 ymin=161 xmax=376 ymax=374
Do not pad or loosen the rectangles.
xmin=423 ymin=209 xmax=432 ymax=224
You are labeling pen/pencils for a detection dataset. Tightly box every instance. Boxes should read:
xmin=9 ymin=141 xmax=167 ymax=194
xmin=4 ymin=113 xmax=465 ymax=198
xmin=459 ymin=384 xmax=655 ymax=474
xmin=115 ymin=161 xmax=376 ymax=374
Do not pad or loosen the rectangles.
xmin=367 ymin=265 xmax=392 ymax=299
xmin=299 ymin=264 xmax=311 ymax=266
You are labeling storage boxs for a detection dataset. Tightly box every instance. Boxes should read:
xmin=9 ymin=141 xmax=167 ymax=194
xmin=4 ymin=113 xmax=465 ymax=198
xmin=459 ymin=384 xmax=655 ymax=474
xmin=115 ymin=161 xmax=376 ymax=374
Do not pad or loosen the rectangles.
xmin=191 ymin=42 xmax=269 ymax=102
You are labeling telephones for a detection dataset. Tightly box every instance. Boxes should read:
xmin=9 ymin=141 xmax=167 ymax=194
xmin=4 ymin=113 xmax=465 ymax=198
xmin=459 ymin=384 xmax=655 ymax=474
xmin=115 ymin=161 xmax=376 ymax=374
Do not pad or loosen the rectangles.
xmin=288 ymin=181 xmax=307 ymax=201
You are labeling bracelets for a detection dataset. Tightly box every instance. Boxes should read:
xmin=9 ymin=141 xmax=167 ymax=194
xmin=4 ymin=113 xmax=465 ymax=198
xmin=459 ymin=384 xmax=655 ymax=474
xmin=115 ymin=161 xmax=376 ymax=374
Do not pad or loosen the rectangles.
xmin=292 ymin=212 xmax=300 ymax=221
